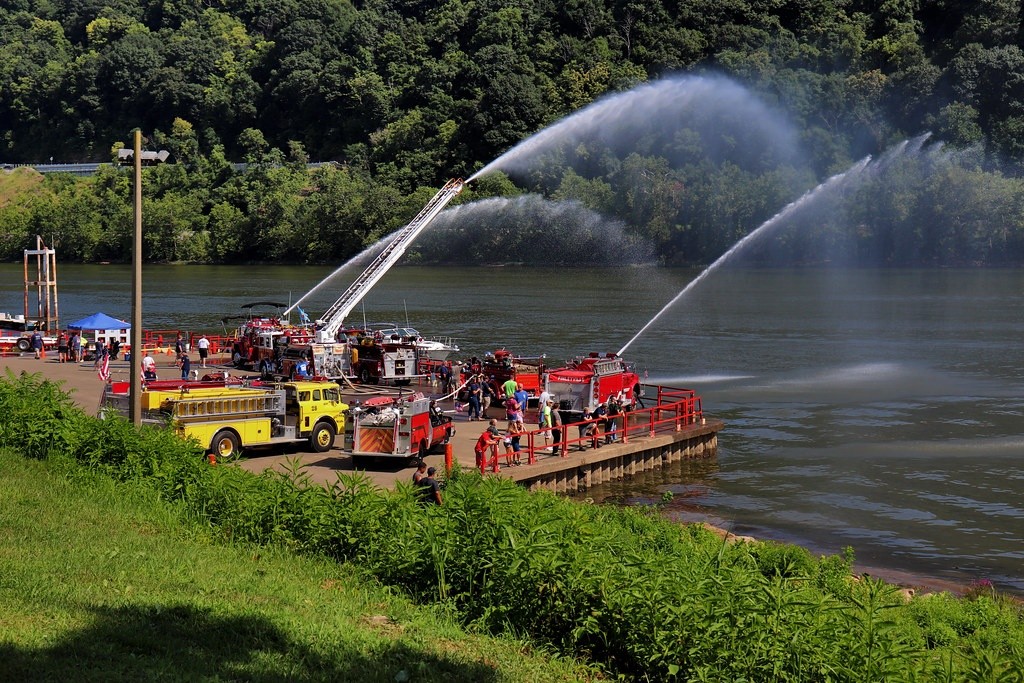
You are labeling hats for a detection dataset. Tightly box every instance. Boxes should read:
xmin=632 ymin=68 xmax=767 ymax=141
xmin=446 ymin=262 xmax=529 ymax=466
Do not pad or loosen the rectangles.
xmin=546 ymin=399 xmax=554 ymax=404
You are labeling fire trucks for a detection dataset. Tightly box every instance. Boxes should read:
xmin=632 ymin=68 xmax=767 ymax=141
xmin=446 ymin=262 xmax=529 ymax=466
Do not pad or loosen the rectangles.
xmin=231 ymin=176 xmax=441 ymax=387
xmin=97 ymin=372 xmax=351 ymax=465
xmin=536 ymin=352 xmax=648 ymax=416
xmin=341 ymin=391 xmax=453 ymax=468
xmin=441 ymin=349 xmax=549 ymax=407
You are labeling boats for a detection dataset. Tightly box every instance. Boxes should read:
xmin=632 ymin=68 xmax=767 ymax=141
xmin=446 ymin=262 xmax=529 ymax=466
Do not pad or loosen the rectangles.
xmin=376 ymin=325 xmax=461 ymax=363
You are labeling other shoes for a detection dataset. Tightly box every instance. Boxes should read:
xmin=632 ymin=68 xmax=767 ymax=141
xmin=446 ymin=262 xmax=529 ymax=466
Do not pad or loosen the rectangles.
xmin=579 ymin=448 xmax=587 ymax=451
xmin=551 ymin=453 xmax=560 ymax=456
xmin=507 ymin=463 xmax=514 ymax=467
xmin=198 ymin=366 xmax=208 ymax=369
xmin=71 ymin=359 xmax=84 ymax=363
xmin=35 ymin=357 xmax=40 ymax=360
xmin=514 ymin=461 xmax=521 ymax=465
xmin=545 ymin=446 xmax=553 ymax=450
xmin=60 ymin=362 xmax=67 ymax=364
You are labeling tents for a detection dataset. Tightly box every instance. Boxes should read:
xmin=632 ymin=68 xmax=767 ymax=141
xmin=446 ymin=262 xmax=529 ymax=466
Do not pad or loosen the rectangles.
xmin=66 ymin=312 xmax=131 ymax=361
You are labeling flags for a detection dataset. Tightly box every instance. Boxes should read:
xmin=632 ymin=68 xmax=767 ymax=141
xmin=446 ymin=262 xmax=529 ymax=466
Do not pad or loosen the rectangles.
xmin=140 ymin=361 xmax=146 ymax=385
xmin=97 ymin=353 xmax=110 ymax=381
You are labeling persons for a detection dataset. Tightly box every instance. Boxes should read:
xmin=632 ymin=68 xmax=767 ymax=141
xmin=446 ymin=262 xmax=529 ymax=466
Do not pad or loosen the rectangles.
xmin=58 ymin=330 xmax=120 ymax=363
xmin=31 ymin=327 xmax=44 ymax=359
xmin=412 ymin=461 xmax=442 ymax=513
xmin=140 ymin=352 xmax=156 ymax=382
xmin=431 ymin=354 xmax=649 ymax=471
xmin=197 ymin=334 xmax=210 ymax=369
xmin=176 ymin=332 xmax=190 ymax=380
xmin=296 ymin=355 xmax=310 ymax=377
xmin=429 ymin=399 xmax=445 ymax=427
